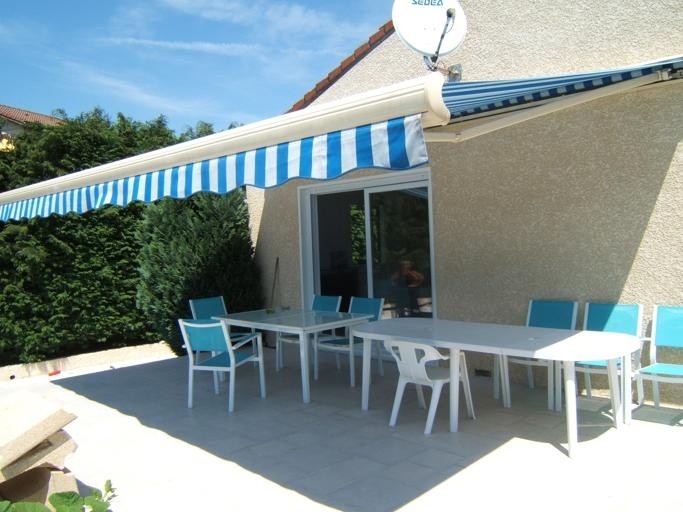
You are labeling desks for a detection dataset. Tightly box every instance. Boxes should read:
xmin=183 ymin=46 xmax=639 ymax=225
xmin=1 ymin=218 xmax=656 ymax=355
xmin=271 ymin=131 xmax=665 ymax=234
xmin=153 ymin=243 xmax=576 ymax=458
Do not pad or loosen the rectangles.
xmin=212 ymin=307 xmax=375 ymax=404
xmin=346 ymin=314 xmax=642 ymax=461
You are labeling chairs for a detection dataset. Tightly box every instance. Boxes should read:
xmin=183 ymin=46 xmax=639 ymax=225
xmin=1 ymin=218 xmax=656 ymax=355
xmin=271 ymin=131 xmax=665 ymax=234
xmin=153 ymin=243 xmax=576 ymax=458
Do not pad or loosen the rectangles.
xmin=189 ymin=295 xmax=257 ymax=394
xmin=635 ymin=304 xmax=682 ymax=423
xmin=556 ymin=302 xmax=644 ymax=419
xmin=384 ymin=339 xmax=476 ymax=436
xmin=314 ymin=296 xmax=385 ymax=387
xmin=179 ymin=319 xmax=265 ymax=412
xmin=494 ymin=298 xmax=579 ymax=410
xmin=278 ymin=296 xmax=343 ymax=380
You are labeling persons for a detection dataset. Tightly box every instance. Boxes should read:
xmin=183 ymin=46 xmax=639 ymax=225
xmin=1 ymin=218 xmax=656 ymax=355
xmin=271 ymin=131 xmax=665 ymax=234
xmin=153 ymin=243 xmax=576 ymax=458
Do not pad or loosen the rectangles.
xmin=390 ymin=258 xmax=424 ymax=318
